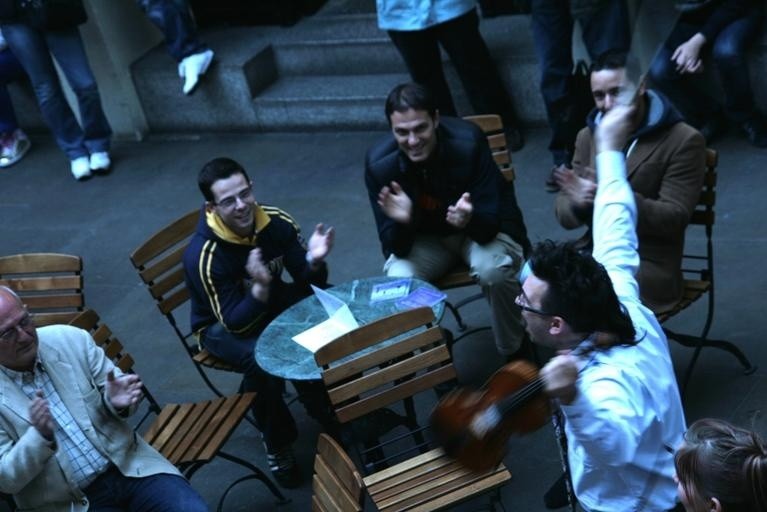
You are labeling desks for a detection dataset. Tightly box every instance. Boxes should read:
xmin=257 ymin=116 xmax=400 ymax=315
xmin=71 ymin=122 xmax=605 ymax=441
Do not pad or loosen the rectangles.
xmin=247 ymin=273 xmax=451 ymax=480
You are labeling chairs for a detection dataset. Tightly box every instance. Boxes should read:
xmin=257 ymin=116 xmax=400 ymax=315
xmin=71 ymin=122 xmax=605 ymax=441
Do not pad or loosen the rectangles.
xmin=420 ymin=114 xmax=519 ymax=350
xmin=125 ymin=202 xmax=301 ymax=435
xmin=309 ymin=431 xmax=367 ymax=512
xmin=0 ymin=251 xmax=86 ymax=333
xmin=59 ymin=307 xmax=294 ymax=512
xmin=305 ymin=303 xmax=514 ymax=511
xmin=640 ymin=147 xmax=760 ymax=416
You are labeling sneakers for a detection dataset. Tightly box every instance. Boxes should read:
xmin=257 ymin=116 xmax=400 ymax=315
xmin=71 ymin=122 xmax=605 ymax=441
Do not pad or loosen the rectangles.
xmin=177 ymin=49 xmax=215 ymax=97
xmin=257 ymin=429 xmax=304 ymax=488
xmin=504 ymin=125 xmax=524 ymax=151
xmin=544 ymin=474 xmax=574 ymax=510
xmin=739 ymin=109 xmax=767 ymax=148
xmin=319 ymin=409 xmax=354 ymax=450
xmin=71 ymin=150 xmax=112 ymax=181
xmin=0 ymin=127 xmax=32 ymax=168
xmin=545 ymin=148 xmax=571 ymax=192
xmin=698 ymin=112 xmax=729 ymax=142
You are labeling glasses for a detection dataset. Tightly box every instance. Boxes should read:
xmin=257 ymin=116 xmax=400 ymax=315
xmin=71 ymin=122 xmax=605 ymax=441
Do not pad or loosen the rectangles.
xmin=0 ymin=315 xmax=34 ymax=342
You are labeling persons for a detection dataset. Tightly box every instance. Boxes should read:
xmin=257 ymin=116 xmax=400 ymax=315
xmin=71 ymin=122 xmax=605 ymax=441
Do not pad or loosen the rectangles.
xmin=181 ymin=157 xmax=335 ymax=477
xmin=137 ymin=0 xmax=215 ymax=95
xmin=364 ymin=2 xmax=766 ymax=512
xmin=0 ymin=0 xmax=113 ymax=181
xmin=0 ymin=31 xmax=31 ymax=169
xmin=0 ymin=285 xmax=211 ymax=512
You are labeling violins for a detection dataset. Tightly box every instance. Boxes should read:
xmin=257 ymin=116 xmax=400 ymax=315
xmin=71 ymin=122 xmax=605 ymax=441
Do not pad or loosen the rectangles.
xmin=428 ymin=332 xmax=608 ymax=475
